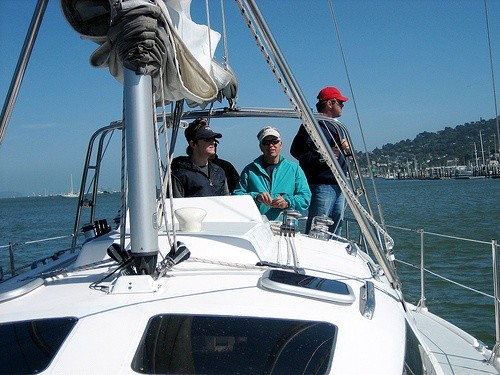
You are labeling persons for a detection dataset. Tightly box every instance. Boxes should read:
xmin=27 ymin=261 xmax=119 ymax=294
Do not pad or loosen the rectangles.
xmin=208 ymin=139 xmax=239 ymax=196
xmin=290 ymin=87 xmax=354 ymax=241
xmin=163 ymin=120 xmax=229 ymax=198
xmin=233 ymin=126 xmax=312 ymax=231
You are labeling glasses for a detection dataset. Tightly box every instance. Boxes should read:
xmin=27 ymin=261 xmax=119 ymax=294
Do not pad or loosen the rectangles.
xmin=262 ymin=139 xmax=279 ymax=146
xmin=200 ymin=137 xmax=215 ymax=142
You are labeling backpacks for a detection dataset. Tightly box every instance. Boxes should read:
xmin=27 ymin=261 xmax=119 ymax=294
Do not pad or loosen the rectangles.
xmin=290 ymin=124 xmax=310 ymax=159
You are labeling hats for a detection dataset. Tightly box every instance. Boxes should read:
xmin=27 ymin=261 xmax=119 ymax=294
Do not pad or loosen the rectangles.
xmin=185 ymin=126 xmax=222 ymax=139
xmin=257 ymin=129 xmax=280 ymax=143
xmin=319 ymin=87 xmax=349 ymax=102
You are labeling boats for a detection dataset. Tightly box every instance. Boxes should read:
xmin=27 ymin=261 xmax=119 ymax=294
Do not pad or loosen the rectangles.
xmin=61 ymin=192 xmax=80 ymax=197
